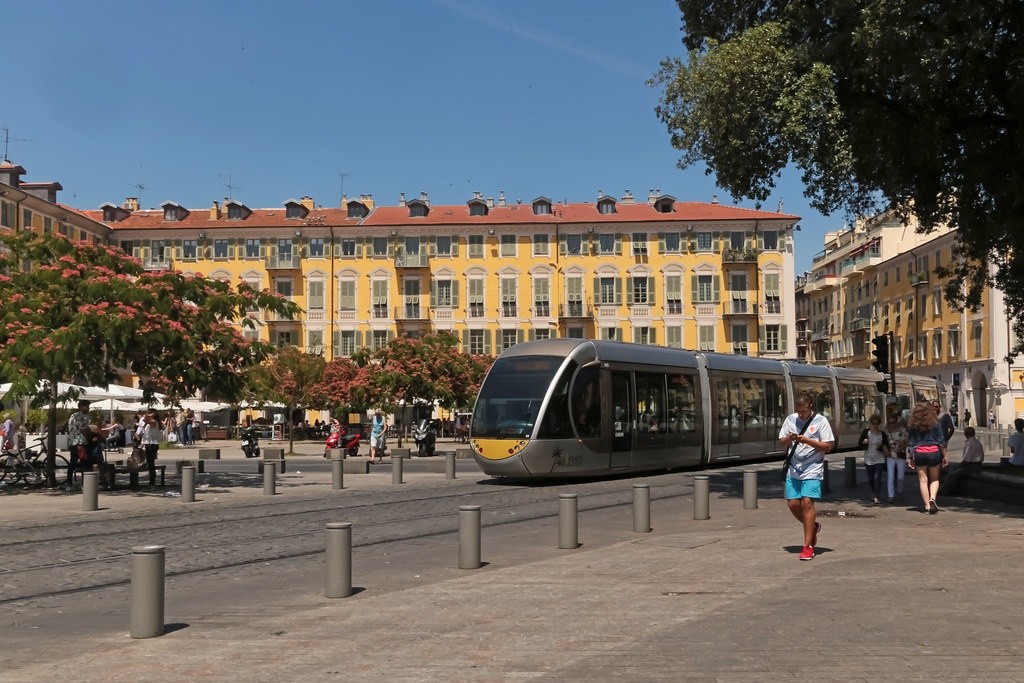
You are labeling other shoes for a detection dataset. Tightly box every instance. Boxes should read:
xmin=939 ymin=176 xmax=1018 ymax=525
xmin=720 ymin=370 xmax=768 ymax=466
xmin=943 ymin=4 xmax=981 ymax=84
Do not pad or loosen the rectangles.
xmin=378 ymin=460 xmax=383 ymax=464
xmin=923 ymin=506 xmax=931 ymax=512
xmin=929 ymin=498 xmax=938 ymax=513
xmin=898 ymin=490 xmax=904 ymax=497
xmin=887 ymin=498 xmax=895 ymax=503
xmin=67 ymin=485 xmax=79 ymax=491
xmin=874 ymin=498 xmax=882 ymax=503
xmin=178 ymin=444 xmax=185 ymax=447
xmin=369 ymin=460 xmax=374 ymax=465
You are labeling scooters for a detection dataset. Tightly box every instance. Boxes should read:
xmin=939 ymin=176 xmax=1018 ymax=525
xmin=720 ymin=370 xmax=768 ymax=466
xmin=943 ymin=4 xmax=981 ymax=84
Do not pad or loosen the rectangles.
xmin=240 ymin=424 xmax=260 ymax=458
xmin=323 ymin=425 xmax=360 ymax=457
xmin=98 ymin=429 xmax=125 ymax=454
xmin=412 ymin=419 xmax=437 ymax=457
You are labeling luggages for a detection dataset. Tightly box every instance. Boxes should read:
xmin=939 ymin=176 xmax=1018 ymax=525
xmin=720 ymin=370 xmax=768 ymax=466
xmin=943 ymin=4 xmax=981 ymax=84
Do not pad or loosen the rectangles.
xmin=99 ymin=436 xmax=115 ymax=491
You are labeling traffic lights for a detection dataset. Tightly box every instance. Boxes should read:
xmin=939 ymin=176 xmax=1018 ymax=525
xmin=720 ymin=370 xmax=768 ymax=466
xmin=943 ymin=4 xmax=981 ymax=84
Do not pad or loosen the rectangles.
xmin=872 ymin=336 xmax=888 ymax=374
xmin=875 ymin=381 xmax=888 ymax=392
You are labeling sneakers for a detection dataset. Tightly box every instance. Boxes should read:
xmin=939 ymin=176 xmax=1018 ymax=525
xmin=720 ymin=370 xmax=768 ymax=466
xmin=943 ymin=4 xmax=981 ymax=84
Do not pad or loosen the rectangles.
xmin=799 ymin=545 xmax=815 ymax=559
xmin=812 ymin=522 xmax=822 ymax=547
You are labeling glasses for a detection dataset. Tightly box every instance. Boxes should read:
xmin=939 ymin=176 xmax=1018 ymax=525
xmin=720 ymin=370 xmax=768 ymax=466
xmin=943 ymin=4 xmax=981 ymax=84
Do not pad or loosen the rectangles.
xmin=933 ymin=405 xmax=939 ymax=408
xmin=887 ymin=421 xmax=896 ymax=424
xmin=871 ymin=422 xmax=879 ymax=425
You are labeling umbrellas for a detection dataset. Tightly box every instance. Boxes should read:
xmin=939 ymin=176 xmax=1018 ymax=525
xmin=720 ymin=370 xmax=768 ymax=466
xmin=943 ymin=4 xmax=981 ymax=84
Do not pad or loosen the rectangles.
xmin=0 ymin=378 xmax=306 ymax=439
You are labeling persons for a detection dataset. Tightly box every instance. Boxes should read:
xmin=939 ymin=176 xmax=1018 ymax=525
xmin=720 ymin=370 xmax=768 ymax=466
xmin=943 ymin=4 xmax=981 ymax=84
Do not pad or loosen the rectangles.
xmin=778 ymin=395 xmax=835 ymax=560
xmin=297 ymin=417 xmax=341 ymax=437
xmin=368 ymin=408 xmax=387 ymax=465
xmin=858 ymin=402 xmax=995 ymax=515
xmin=1004 ymin=418 xmax=1024 ymax=466
xmin=410 ymin=412 xmax=469 ymax=444
xmin=0 ymin=400 xmax=195 ymax=491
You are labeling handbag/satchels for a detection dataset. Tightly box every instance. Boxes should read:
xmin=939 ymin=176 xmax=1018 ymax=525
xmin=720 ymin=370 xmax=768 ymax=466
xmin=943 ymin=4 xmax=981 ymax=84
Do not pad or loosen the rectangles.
xmin=132 ymin=443 xmax=148 ymax=471
xmin=781 ymin=458 xmax=790 ymax=482
xmin=167 ymin=431 xmax=177 ymax=443
xmin=897 ymin=450 xmax=906 ymax=458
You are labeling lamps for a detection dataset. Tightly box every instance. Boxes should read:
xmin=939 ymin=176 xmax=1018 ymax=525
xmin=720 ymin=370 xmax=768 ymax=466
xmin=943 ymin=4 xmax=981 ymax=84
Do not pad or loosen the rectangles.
xmin=794 ymin=225 xmax=801 ymax=231
xmin=489 ymin=229 xmax=495 ymax=236
xmin=391 ymin=231 xmax=397 ymax=236
xmin=295 ymin=232 xmax=301 ymax=237
xmin=199 ymin=233 xmax=205 ymax=238
xmin=1 ymin=190 xmax=11 ymax=196
xmin=63 ymin=217 xmax=71 ymax=222
xmin=786 ymin=224 xmax=792 ymax=230
xmin=687 ymin=226 xmax=693 ymax=232
xmin=588 ymin=228 xmax=594 ymax=233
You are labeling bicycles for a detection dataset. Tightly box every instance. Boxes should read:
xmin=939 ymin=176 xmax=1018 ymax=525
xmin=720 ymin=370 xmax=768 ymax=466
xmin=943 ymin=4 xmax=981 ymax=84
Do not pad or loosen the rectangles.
xmin=0 ymin=440 xmax=48 ymax=486
xmin=4 ymin=435 xmax=71 ymax=485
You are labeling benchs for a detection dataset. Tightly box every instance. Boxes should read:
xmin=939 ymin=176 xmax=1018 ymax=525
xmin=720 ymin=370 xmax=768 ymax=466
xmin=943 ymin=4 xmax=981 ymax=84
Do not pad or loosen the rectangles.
xmin=939 ymin=462 xmax=1024 ymax=507
xmin=74 ymin=465 xmax=165 ymax=488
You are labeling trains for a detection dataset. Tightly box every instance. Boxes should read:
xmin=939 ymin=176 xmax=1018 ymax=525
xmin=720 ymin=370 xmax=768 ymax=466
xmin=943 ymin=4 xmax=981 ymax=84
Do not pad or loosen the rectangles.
xmin=469 ymin=338 xmax=947 ymax=481
